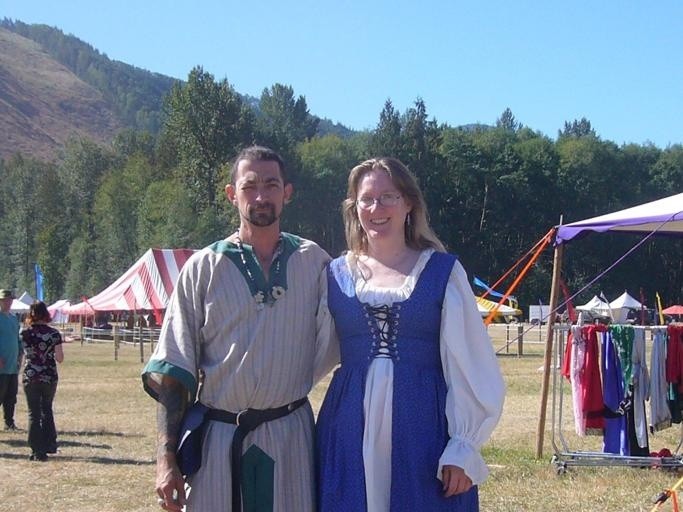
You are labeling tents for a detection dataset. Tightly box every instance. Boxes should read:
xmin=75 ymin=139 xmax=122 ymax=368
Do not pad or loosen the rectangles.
xmin=605 ymin=291 xmax=647 ymax=325
xmin=575 ymin=295 xmax=609 ymax=323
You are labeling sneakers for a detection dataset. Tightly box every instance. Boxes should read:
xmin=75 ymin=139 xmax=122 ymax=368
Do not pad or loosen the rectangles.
xmin=30 ymin=455 xmax=48 ymax=461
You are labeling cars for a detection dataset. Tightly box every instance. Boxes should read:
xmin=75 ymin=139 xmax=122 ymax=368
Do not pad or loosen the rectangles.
xmin=560 ymin=308 xmax=611 ymax=325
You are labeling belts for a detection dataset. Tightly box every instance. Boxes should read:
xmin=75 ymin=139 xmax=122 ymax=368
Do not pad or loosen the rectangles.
xmin=195 ymin=397 xmax=308 ymax=512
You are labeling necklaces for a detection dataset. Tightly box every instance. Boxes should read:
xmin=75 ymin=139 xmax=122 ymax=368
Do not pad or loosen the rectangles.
xmin=234 ymin=226 xmax=286 ymax=311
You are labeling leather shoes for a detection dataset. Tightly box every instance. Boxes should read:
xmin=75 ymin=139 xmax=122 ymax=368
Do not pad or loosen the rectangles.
xmin=4 ymin=427 xmax=25 ymax=434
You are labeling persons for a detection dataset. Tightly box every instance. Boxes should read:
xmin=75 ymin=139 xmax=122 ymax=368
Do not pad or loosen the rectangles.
xmin=196 ymin=155 xmax=507 ymax=512
xmin=0 ymin=288 xmax=26 ymax=433
xmin=18 ymin=298 xmax=63 ymax=462
xmin=139 ymin=146 xmax=342 ymax=512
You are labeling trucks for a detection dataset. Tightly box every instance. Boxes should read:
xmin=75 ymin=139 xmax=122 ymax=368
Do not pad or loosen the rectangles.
xmin=528 ymin=305 xmax=560 ymax=325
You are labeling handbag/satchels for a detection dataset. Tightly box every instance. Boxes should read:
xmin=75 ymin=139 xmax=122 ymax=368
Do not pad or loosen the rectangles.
xmin=175 ymin=407 xmax=208 ymax=475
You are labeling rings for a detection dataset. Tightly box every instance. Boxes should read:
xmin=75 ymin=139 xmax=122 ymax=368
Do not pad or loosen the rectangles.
xmin=157 ymin=495 xmax=165 ymax=506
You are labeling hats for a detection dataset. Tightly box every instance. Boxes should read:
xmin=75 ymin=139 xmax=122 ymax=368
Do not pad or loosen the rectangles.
xmin=0 ymin=290 xmax=16 ymax=300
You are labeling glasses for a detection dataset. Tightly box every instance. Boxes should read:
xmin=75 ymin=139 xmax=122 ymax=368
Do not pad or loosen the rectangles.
xmin=355 ymin=193 xmax=404 ymax=211
